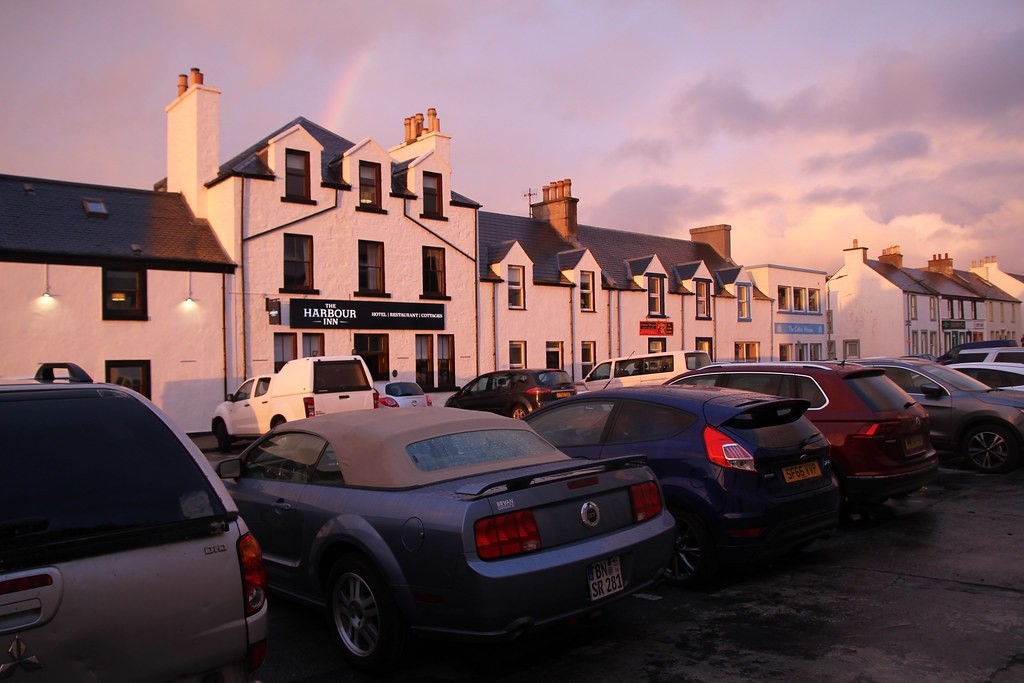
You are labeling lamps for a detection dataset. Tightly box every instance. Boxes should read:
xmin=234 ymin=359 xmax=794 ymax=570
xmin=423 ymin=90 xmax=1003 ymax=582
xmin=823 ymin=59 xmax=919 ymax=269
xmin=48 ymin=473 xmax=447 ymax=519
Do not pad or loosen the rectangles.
xmin=112 ymin=293 xmax=126 ymax=302
xmin=186 ymin=271 xmax=193 ymax=302
xmin=268 ymin=297 xmax=280 ymax=317
xmin=42 ymin=265 xmax=51 ymax=297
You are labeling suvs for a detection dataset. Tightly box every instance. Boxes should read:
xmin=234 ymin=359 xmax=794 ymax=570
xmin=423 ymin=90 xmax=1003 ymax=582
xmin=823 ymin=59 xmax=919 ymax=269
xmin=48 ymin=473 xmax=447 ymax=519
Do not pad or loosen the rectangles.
xmin=0 ymin=361 xmax=269 ymax=683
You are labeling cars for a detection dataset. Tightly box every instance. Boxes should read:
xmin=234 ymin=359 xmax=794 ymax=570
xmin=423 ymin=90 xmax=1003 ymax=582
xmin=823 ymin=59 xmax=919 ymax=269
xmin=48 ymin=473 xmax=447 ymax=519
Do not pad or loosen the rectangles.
xmin=211 ymin=355 xmax=375 ymax=454
xmin=209 ymin=406 xmax=678 ymax=673
xmin=444 ymin=368 xmax=577 ymax=419
xmin=837 ymin=357 xmax=1024 ymax=475
xmin=522 ymin=384 xmax=844 ymax=590
xmin=374 ymin=381 xmax=432 ymax=408
xmin=944 ymin=361 xmax=1024 ymax=393
xmin=661 ymin=361 xmax=940 ymax=526
xmin=899 ymin=354 xmax=936 ymax=360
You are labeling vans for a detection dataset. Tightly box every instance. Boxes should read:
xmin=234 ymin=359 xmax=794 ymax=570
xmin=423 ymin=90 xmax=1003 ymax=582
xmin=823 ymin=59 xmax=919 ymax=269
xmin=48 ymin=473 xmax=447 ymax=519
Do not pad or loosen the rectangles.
xmin=577 ymin=350 xmax=712 ymax=392
xmin=934 ymin=339 xmax=1018 ymax=366
xmin=954 ymin=347 xmax=1024 ymax=364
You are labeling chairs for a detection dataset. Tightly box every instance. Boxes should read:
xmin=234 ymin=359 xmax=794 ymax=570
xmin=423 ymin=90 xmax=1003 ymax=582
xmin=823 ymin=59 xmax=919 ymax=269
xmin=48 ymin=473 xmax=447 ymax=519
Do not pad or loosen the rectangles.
xmin=279 ymin=448 xmax=332 ymax=478
xmin=616 ymin=361 xmax=649 ymax=377
xmin=497 ymin=378 xmax=507 ymax=388
